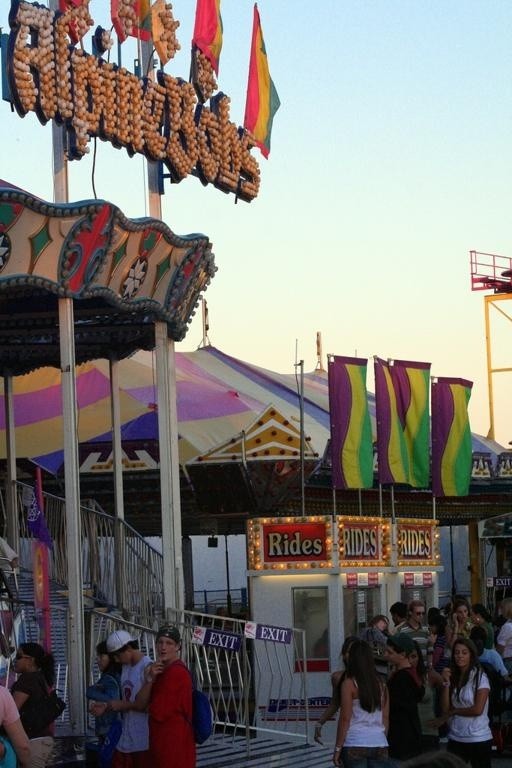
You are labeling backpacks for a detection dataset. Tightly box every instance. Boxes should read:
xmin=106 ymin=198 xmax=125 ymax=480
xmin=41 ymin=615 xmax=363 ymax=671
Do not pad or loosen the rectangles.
xmin=177 ymin=663 xmax=212 ymax=745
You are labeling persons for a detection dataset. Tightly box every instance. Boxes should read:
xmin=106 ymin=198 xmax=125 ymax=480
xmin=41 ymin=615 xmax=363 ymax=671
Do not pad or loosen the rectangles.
xmin=314 ymin=593 xmax=511 ymax=764
xmin=0 ymin=535 xmax=19 ymax=571
xmin=0 ymin=620 xmax=197 ymax=765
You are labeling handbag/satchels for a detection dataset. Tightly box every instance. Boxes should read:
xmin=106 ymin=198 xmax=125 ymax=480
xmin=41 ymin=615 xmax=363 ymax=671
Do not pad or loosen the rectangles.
xmin=24 ymin=675 xmax=65 ymax=734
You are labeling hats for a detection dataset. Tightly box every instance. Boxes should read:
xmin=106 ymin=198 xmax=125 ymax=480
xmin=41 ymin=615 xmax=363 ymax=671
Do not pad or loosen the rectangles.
xmin=86 ymin=673 xmax=120 ymax=734
xmin=156 ymin=626 xmax=182 ymax=650
xmin=106 ymin=631 xmax=141 ymax=653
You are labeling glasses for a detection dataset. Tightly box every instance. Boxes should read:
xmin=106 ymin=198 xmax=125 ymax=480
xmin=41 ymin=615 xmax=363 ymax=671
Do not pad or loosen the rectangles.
xmin=15 ymin=652 xmax=32 ymax=660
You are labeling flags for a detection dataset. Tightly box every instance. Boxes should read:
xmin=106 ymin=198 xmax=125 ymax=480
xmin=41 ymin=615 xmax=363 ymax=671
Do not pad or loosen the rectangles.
xmin=326 ymin=355 xmax=474 ymax=500
xmin=190 ymin=1 xmax=226 ymax=80
xmin=22 ymin=482 xmax=55 ymax=550
xmin=58 ymin=0 xmax=95 ymax=47
xmin=109 ymin=0 xmax=155 ymax=51
xmin=241 ymin=8 xmax=282 ymax=161
